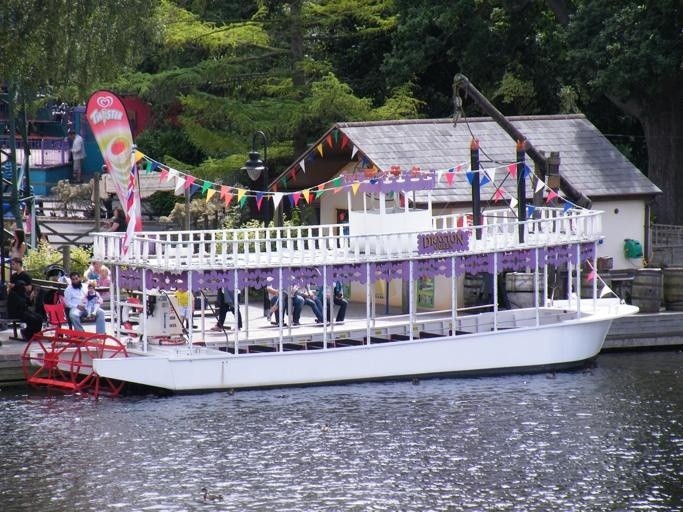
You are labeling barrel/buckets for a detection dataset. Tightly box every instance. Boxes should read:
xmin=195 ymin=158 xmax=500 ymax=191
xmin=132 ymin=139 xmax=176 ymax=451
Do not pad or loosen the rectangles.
xmin=664 ymin=267 xmax=683 ymax=310
xmin=583 ymin=272 xmax=612 ymax=298
xmin=631 ymin=271 xmax=664 ymax=312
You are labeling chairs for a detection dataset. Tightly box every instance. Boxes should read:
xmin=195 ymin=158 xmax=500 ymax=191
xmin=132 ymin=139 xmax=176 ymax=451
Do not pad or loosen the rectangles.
xmin=120 ymin=289 xmax=241 ymax=333
xmin=1 ymin=276 xmax=111 ymax=342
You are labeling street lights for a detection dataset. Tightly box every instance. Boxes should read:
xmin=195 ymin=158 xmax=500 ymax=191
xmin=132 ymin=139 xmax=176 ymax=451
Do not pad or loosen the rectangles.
xmin=239 ymin=127 xmax=279 ymax=318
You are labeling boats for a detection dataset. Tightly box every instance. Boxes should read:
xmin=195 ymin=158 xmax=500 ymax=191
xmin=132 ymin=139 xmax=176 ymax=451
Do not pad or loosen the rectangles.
xmin=18 ymin=69 xmax=643 ymax=393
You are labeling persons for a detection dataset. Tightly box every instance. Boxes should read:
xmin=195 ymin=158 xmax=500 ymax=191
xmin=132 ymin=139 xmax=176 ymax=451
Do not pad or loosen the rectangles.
xmin=66 ymin=129 xmax=86 ymax=182
xmin=173 ymin=285 xmax=195 ymax=330
xmin=263 ymin=273 xmax=348 ymax=327
xmin=8 ymin=278 xmax=43 ymax=341
xmin=9 ymin=226 xmax=26 ymax=273
xmin=65 ymin=121 xmax=72 ymax=160
xmin=83 ymin=280 xmax=104 ymax=318
xmin=62 ymin=271 xmax=108 ymax=339
xmin=22 ymin=206 xmax=32 ymax=233
xmin=5 ymin=257 xmax=32 ymax=294
xmin=229 ymin=289 xmax=243 ymax=330
xmin=106 ymin=205 xmax=129 ymax=233
xmin=213 ymin=287 xmax=229 ymax=332
xmin=85 ymin=255 xmax=113 ymax=290
xmin=97 ymin=266 xmax=112 ymax=300
